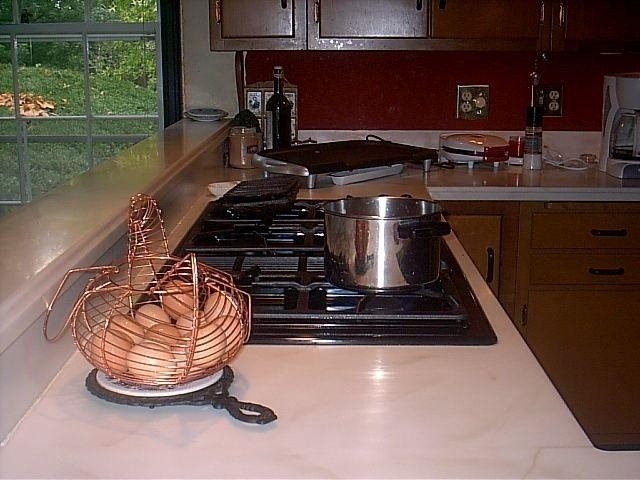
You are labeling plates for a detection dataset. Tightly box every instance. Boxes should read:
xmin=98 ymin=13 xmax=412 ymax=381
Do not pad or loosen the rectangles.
xmin=183 ymin=108 xmax=228 ymax=122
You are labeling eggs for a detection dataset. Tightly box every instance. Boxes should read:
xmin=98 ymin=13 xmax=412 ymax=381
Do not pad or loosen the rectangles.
xmin=92 ymin=278 xmax=242 ymax=384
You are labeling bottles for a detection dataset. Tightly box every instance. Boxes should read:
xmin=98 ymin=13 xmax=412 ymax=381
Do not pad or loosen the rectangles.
xmin=508 ymin=136 xmax=525 ymax=166
xmin=525 ymin=107 xmax=543 ymax=170
xmin=264 ymin=66 xmax=291 ymax=150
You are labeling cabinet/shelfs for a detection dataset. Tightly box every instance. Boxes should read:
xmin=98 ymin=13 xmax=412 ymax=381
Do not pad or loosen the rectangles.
xmin=433 ymin=200 xmax=518 ymax=326
xmin=207 ymin=0 xmax=308 ymax=52
xmin=516 ymin=201 xmax=640 ymax=451
xmin=552 ymin=0 xmax=640 ymax=52
xmin=308 ymin=1 xmax=552 ymax=52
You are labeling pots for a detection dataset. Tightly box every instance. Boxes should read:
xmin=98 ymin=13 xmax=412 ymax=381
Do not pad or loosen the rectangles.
xmin=321 ymin=197 xmax=452 ymax=294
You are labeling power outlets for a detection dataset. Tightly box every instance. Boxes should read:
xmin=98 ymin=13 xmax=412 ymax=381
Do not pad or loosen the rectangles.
xmin=456 ymin=84 xmax=491 ymax=120
xmin=531 ymin=83 xmax=564 ymax=117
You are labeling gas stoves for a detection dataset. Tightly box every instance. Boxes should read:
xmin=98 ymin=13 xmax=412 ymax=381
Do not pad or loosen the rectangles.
xmin=124 ymin=193 xmax=498 ymax=346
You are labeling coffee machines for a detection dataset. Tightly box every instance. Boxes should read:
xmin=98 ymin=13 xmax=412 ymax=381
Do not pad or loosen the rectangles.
xmin=598 ymin=75 xmax=640 ymax=180
